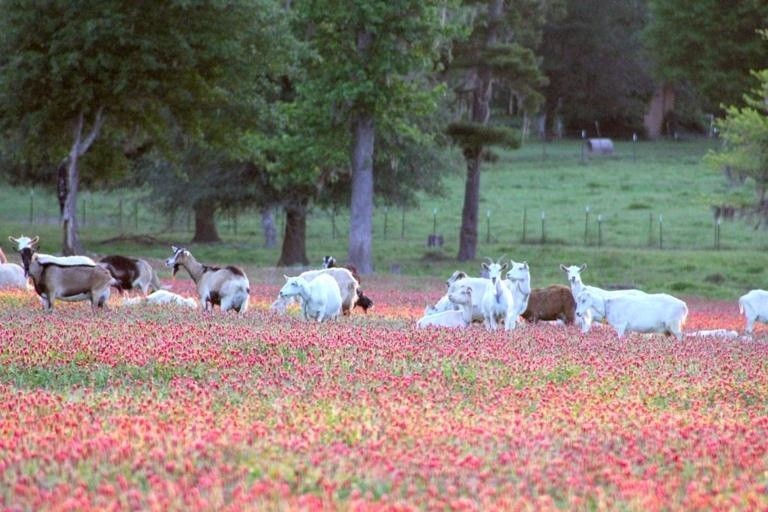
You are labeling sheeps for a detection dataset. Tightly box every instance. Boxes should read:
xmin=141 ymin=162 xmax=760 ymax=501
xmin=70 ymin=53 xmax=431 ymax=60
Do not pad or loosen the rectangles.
xmin=0 ymin=235 xmax=250 ymax=319
xmin=270 ymin=255 xmax=374 ymax=323
xmin=738 ymin=290 xmax=768 ymax=342
xmin=416 ymin=253 xmax=689 ymax=341
